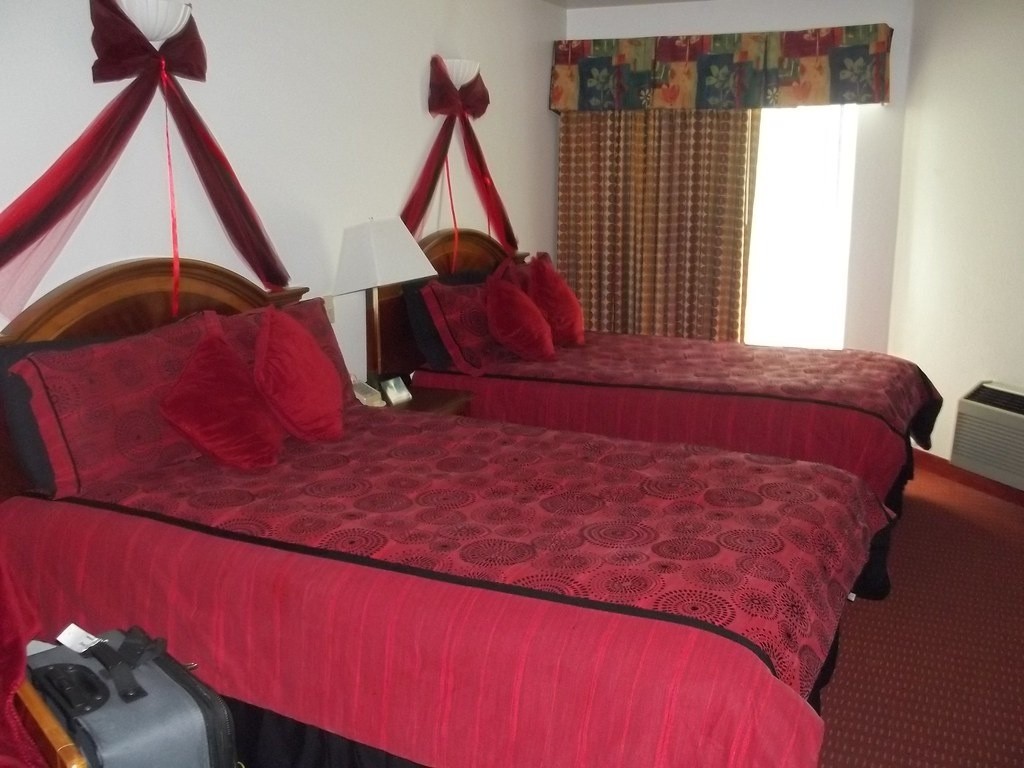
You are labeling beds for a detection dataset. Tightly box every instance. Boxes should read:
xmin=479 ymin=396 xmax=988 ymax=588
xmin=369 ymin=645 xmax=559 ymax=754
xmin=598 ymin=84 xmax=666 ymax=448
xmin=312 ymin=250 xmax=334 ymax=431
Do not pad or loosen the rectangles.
xmin=0 ymin=256 xmax=897 ymax=768
xmin=365 ymin=227 xmax=943 ymax=600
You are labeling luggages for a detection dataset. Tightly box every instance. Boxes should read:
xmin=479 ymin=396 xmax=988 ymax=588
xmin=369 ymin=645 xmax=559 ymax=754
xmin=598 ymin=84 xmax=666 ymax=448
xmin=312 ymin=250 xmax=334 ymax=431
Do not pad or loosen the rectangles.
xmin=12 ymin=625 xmax=238 ymax=768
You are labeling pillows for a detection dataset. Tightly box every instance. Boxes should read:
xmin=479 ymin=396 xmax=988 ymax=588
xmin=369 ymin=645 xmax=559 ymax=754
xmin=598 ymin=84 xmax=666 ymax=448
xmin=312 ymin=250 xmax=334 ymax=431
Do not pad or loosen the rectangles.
xmin=215 ymin=297 xmax=364 ymax=440
xmin=159 ymin=325 xmax=289 ymax=475
xmin=421 ymin=258 xmax=524 ymax=377
xmin=525 ymin=259 xmax=586 ymax=347
xmin=250 ymin=305 xmax=344 ymax=448
xmin=1 ymin=310 xmax=243 ymax=500
xmin=481 ymin=275 xmax=556 ymax=362
